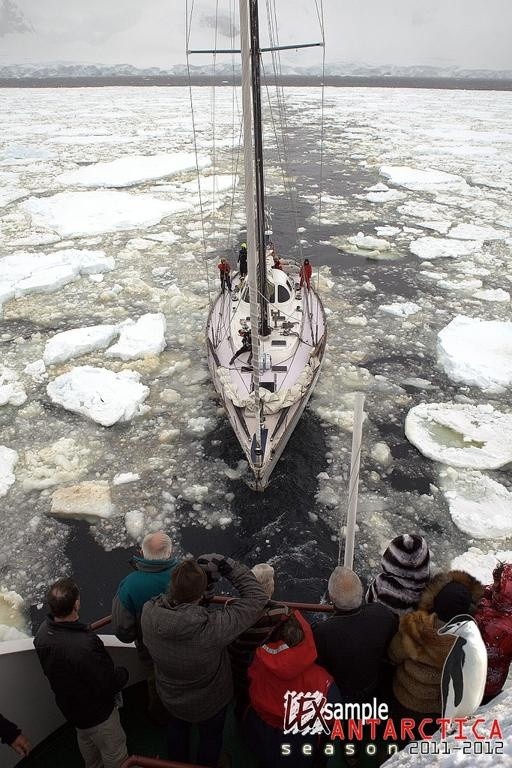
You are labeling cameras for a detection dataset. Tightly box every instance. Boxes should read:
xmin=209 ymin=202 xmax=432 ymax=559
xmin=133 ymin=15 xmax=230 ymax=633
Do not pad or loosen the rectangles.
xmin=200 ymin=560 xmax=218 ymax=590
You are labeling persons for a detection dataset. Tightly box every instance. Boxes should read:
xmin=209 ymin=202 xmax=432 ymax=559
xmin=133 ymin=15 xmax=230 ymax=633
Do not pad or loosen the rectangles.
xmin=218 ymin=259 xmax=232 ymax=294
xmin=237 ymin=247 xmax=247 ymax=277
xmin=298 ymin=259 xmax=312 ymax=292
xmin=33 ymin=579 xmax=139 ymax=768
xmin=0 ymin=713 xmax=32 ymax=756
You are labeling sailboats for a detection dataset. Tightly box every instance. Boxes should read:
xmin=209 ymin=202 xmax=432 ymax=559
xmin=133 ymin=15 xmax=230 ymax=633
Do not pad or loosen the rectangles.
xmin=181 ymin=0 xmax=331 ymax=486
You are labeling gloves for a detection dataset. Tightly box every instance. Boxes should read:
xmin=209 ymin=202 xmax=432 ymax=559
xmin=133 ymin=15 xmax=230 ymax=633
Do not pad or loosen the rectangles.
xmin=197 ymin=553 xmax=226 ymax=572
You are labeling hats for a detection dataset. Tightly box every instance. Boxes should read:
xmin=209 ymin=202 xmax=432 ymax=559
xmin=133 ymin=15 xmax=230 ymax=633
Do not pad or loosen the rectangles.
xmin=221 ymin=258 xmax=225 ymax=263
